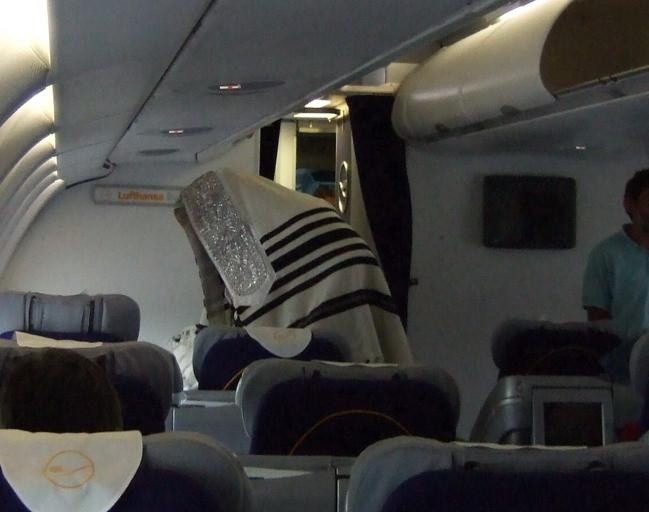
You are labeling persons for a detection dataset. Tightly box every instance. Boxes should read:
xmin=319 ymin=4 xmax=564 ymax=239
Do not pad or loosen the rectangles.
xmin=0 ymin=347 xmax=124 ymax=433
xmin=584 ymin=167 xmax=649 ymax=342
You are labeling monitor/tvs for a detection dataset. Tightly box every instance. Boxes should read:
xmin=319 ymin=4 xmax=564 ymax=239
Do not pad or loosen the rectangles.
xmin=482 ymin=173 xmax=577 ymax=250
xmin=531 ymin=386 xmax=616 ymax=448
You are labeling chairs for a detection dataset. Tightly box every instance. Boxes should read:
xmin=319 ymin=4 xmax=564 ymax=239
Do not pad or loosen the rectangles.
xmin=345 ymin=436 xmax=649 ymax=512
xmin=0 ymin=428 xmax=257 ymax=511
xmin=192 ymin=325 xmax=353 ymax=391
xmin=234 ymin=358 xmax=461 ymax=457
xmin=469 ymin=319 xmax=624 ymax=445
xmin=0 ymin=342 xmax=183 ymax=434
xmin=0 ymin=291 xmax=140 ymax=341
